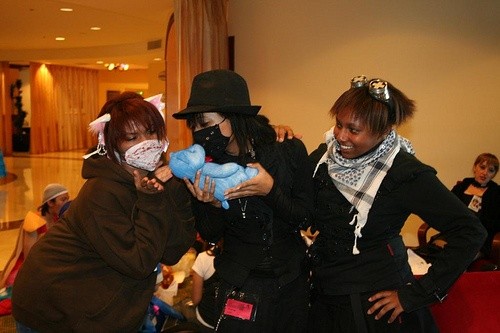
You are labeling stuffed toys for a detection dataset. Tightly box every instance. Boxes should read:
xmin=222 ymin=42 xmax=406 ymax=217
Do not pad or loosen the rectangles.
xmin=168 ymin=144 xmax=259 ymax=210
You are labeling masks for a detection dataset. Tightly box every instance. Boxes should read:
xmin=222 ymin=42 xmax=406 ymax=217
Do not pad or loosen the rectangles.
xmin=192 ymin=118 xmax=234 ymax=157
xmin=125 ymin=136 xmax=170 ymax=171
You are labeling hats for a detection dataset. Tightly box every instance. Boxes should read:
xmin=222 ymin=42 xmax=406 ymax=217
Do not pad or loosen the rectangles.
xmin=41 ymin=184 xmax=68 ymax=204
xmin=172 ymin=70 xmax=262 ymax=120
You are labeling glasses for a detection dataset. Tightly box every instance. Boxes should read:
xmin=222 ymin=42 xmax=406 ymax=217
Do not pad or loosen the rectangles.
xmin=351 ymin=75 xmax=393 ymax=109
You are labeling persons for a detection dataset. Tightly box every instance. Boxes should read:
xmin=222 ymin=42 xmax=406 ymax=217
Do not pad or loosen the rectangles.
xmin=0 ymin=184 xmax=70 ymax=315
xmin=271 ymin=76 xmax=486 ymax=333
xmin=13 ymin=92 xmax=197 ymax=333
xmin=413 ymin=153 xmax=500 ymax=272
xmin=172 ymin=70 xmax=331 ymax=333
